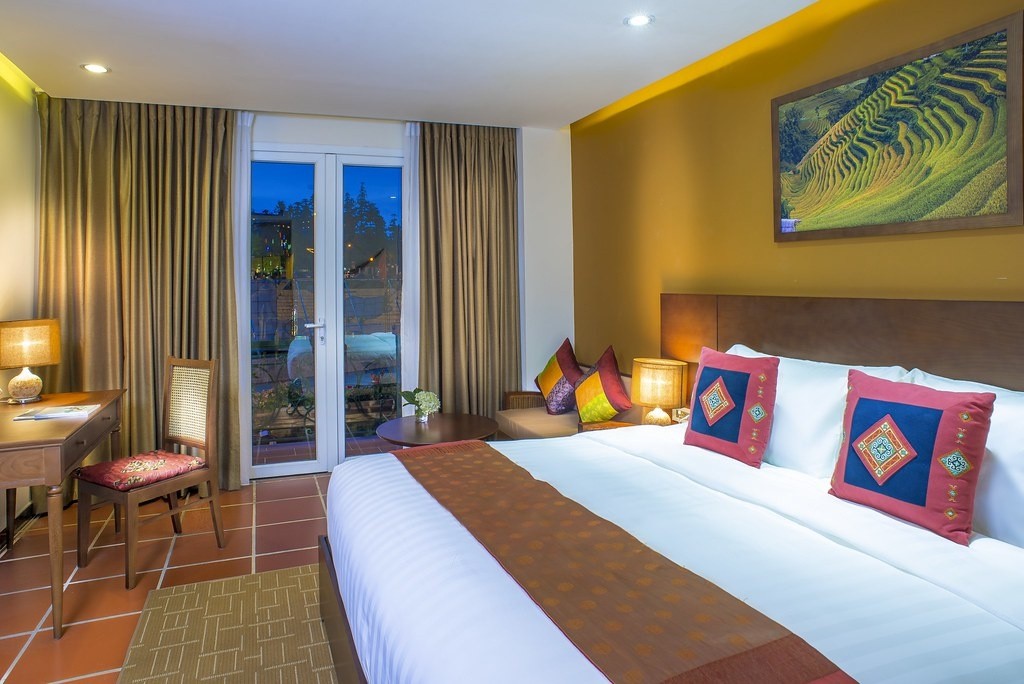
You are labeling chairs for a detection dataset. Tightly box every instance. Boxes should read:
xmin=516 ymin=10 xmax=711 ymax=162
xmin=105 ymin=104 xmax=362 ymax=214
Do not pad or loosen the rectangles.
xmin=68 ymin=353 xmax=227 ymax=590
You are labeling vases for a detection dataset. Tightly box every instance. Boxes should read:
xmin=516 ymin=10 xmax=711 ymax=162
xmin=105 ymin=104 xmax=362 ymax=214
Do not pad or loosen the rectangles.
xmin=415 ymin=405 xmax=429 ymax=422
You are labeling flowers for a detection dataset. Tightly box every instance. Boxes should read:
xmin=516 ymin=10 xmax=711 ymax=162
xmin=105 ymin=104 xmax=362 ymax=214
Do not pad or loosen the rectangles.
xmin=400 ymin=387 xmax=441 ymax=417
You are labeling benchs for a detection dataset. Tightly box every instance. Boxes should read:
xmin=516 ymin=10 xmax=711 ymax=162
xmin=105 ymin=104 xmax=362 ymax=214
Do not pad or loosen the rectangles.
xmin=496 ymin=363 xmax=642 ymax=442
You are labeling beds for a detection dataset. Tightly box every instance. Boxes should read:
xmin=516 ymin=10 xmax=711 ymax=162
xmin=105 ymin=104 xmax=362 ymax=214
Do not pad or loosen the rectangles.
xmin=287 ymin=333 xmax=398 ymax=393
xmin=316 ymin=293 xmax=1024 ymax=684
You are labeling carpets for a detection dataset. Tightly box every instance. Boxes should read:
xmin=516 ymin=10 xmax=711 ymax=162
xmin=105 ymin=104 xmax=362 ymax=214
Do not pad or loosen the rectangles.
xmin=114 ymin=561 xmax=337 ymax=684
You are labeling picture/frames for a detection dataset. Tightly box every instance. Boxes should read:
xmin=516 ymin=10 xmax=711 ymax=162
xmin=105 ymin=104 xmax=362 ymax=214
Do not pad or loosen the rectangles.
xmin=770 ymin=9 xmax=1023 ymax=243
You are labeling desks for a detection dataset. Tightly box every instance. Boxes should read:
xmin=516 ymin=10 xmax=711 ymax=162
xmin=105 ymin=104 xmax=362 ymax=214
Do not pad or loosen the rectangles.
xmin=375 ymin=414 xmax=499 ymax=447
xmin=1 ymin=386 xmax=126 ymax=639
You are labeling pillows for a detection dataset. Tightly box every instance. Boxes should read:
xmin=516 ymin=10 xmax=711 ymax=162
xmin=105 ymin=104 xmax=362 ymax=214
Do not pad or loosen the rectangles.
xmin=826 ymin=370 xmax=999 ymax=550
xmin=721 ymin=341 xmax=908 ymax=474
xmin=574 ymin=345 xmax=631 ymax=422
xmin=682 ymin=346 xmax=781 ymax=469
xmin=532 ymin=336 xmax=583 ymax=415
xmin=897 ymin=367 xmax=1024 ymax=549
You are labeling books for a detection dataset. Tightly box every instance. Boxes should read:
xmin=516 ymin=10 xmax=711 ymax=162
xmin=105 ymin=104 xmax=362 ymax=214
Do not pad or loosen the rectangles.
xmin=13 ymin=404 xmax=101 ymax=420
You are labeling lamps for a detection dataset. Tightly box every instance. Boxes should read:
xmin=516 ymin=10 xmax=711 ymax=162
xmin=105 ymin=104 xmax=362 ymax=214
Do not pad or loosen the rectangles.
xmin=0 ymin=317 xmax=62 ymax=406
xmin=631 ymin=356 xmax=688 ymax=425
